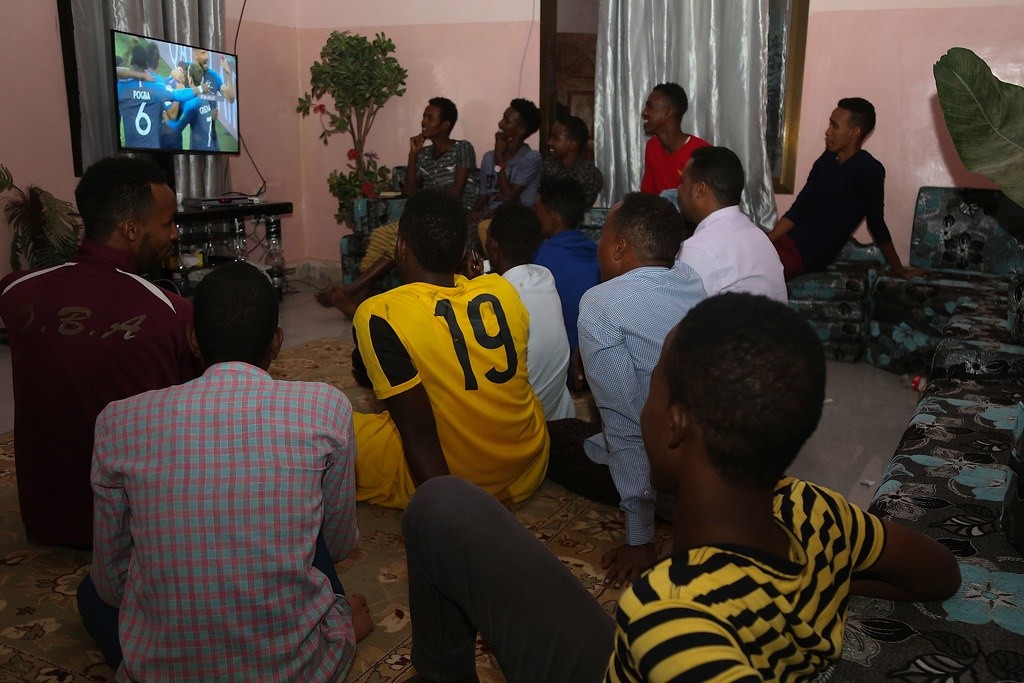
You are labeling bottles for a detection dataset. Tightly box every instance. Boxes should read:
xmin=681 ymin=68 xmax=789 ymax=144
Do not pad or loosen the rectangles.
xmin=900 ymin=373 xmax=926 ymax=392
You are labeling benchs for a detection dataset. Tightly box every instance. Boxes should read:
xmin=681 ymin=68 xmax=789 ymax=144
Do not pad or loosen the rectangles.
xmin=339 ymin=167 xmax=489 ymax=306
xmin=579 ymin=207 xmax=886 ymax=367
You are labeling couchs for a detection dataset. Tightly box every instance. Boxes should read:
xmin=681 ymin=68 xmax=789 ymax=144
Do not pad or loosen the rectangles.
xmin=825 ymin=184 xmax=1023 ymax=683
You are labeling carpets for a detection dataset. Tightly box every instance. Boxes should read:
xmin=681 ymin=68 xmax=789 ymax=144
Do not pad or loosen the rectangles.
xmin=0 ymin=335 xmax=674 ymax=683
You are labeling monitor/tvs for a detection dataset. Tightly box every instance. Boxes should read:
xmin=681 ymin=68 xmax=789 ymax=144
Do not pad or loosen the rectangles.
xmin=110 ymin=29 xmax=240 ymax=156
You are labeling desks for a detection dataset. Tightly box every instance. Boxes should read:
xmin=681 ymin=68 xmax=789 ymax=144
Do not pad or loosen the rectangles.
xmin=148 ymin=200 xmax=293 ymax=303
